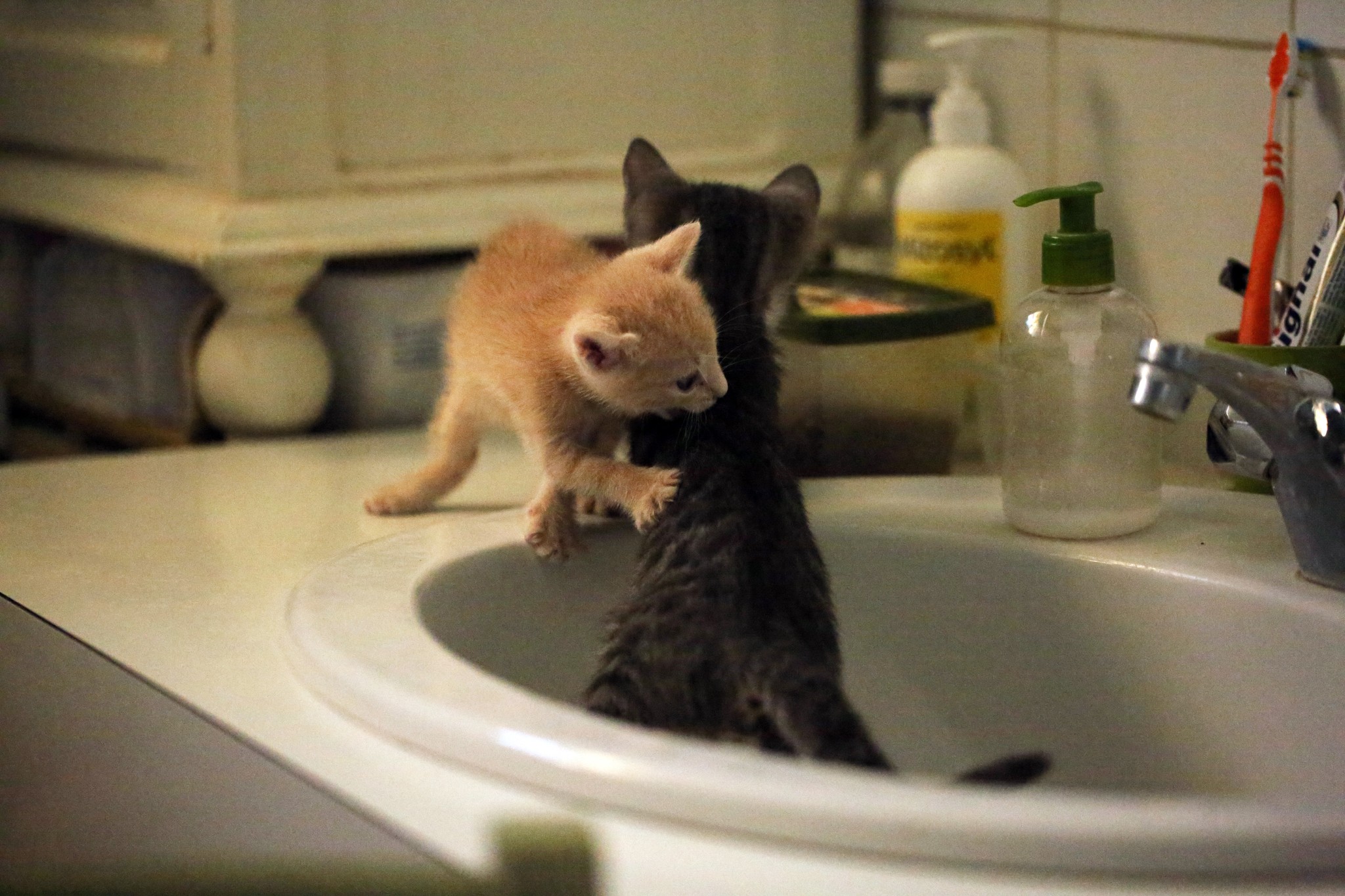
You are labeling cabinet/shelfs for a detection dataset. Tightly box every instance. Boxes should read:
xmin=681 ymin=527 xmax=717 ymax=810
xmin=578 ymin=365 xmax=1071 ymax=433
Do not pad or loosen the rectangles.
xmin=1 ymin=0 xmax=863 ymax=436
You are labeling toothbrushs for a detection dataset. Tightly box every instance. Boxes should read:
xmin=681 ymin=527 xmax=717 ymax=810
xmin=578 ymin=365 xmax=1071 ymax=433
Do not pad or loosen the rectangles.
xmin=1228 ymin=30 xmax=1315 ymax=344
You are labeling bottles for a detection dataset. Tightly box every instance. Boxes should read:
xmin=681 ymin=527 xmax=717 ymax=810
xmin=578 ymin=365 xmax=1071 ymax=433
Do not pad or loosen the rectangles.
xmin=894 ymin=26 xmax=1042 ymax=398
xmin=829 ymin=58 xmax=937 ymax=282
xmin=984 ymin=177 xmax=1169 ymax=542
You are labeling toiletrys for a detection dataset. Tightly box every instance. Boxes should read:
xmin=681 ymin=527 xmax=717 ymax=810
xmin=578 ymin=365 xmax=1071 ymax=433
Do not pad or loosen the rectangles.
xmin=893 ymin=24 xmax=1027 ymax=340
xmin=1267 ymin=183 xmax=1343 ymax=346
xmin=997 ymin=180 xmax=1163 ymax=540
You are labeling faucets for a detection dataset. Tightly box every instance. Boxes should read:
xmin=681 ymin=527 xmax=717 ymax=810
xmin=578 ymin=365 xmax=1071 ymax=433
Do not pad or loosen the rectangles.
xmin=1124 ymin=327 xmax=1343 ymax=585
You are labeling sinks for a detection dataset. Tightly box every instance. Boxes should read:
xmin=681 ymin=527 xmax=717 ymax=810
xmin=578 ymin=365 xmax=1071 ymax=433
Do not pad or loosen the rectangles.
xmin=284 ymin=495 xmax=1343 ymax=879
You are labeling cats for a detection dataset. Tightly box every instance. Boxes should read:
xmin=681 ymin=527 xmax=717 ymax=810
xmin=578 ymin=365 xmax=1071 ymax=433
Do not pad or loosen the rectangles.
xmin=364 ymin=136 xmax=1050 ymax=784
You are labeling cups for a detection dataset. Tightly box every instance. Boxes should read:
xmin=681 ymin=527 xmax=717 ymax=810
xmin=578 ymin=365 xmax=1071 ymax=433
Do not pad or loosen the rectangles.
xmin=1204 ymin=327 xmax=1345 ymax=491
